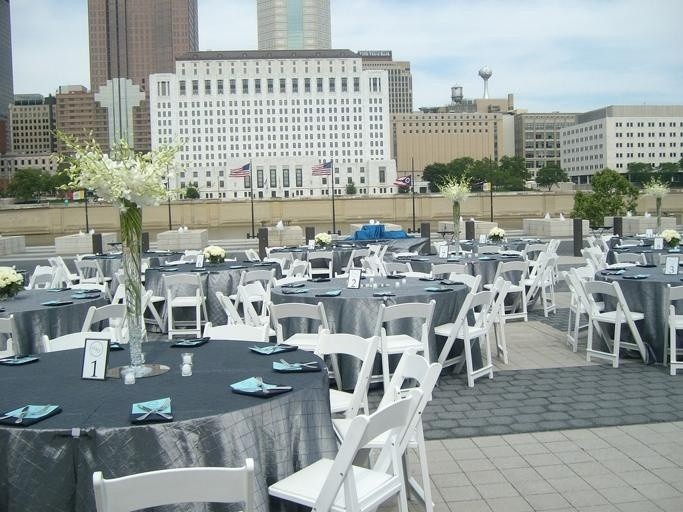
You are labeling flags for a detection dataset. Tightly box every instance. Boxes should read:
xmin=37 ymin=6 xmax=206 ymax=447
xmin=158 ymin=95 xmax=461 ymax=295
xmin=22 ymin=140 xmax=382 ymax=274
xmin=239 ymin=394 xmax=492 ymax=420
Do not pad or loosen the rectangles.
xmin=69 ymin=189 xmax=86 ymax=201
xmin=483 ymin=182 xmax=492 ymax=191
xmin=312 ymin=162 xmax=333 ymax=176
xmin=392 ymin=174 xmax=411 ymax=187
xmin=229 ymin=163 xmax=251 ymax=177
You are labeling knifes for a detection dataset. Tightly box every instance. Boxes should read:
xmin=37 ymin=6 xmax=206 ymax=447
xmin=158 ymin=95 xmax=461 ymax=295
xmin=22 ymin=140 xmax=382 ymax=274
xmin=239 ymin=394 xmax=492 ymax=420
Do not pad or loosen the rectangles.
xmin=14 ymin=402 xmax=29 ymax=426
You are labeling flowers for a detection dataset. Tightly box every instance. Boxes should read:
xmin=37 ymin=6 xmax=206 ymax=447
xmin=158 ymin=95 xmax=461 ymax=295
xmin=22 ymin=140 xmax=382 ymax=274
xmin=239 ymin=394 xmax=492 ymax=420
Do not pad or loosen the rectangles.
xmin=50 ymin=129 xmax=196 ymax=325
xmin=314 ymin=233 xmax=331 ymax=246
xmin=643 ymin=175 xmax=670 ymax=198
xmin=488 ymin=228 xmax=505 ymax=246
xmin=434 ymin=166 xmax=473 ymax=203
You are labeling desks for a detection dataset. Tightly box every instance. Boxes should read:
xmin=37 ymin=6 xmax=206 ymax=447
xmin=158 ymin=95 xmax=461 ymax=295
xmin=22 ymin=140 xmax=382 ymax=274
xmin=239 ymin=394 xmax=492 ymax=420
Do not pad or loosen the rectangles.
xmin=1 ymin=237 xmax=562 ymax=512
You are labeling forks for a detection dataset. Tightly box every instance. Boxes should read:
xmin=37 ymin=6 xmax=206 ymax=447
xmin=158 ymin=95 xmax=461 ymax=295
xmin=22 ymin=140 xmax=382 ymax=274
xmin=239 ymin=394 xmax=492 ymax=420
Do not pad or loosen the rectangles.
xmin=134 ymin=404 xmax=173 ymax=424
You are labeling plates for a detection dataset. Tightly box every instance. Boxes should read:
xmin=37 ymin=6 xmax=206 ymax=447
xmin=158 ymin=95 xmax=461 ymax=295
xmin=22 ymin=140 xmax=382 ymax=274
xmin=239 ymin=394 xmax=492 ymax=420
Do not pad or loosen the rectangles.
xmin=270 ymin=358 xmax=321 ymax=373
xmin=0 ymin=237 xmax=543 ymax=313
xmin=602 ymin=232 xmax=682 ymax=281
xmin=107 ymin=341 xmax=121 ymax=351
xmin=170 ymin=338 xmax=210 ymax=349
xmin=228 ymin=375 xmax=294 ymax=399
xmin=3 ymin=403 xmax=58 ymax=422
xmin=252 ymin=343 xmax=296 ymax=355
xmin=132 ymin=398 xmax=174 ymax=415
xmin=2 ymin=355 xmax=39 ymax=365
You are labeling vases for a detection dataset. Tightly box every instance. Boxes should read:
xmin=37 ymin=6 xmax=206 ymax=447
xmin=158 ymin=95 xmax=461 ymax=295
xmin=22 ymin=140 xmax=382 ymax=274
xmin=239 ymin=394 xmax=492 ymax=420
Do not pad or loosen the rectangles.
xmin=106 ymin=208 xmax=170 ymax=379
xmin=453 ymin=203 xmax=461 ymax=260
xmin=655 ymin=198 xmax=663 ymax=234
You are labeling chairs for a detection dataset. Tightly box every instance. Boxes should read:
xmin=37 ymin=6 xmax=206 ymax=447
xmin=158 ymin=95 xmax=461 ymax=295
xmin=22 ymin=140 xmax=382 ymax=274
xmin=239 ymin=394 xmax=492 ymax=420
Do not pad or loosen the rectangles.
xmin=562 ymin=232 xmax=683 ymax=376
